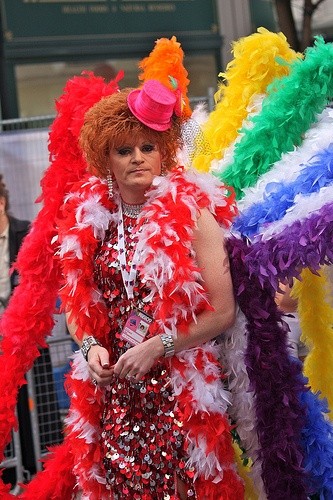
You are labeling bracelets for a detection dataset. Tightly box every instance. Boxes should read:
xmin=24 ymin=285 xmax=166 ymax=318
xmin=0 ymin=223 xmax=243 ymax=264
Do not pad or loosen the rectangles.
xmin=159 ymin=333 xmax=175 ymax=359
xmin=80 ymin=335 xmax=102 ymax=362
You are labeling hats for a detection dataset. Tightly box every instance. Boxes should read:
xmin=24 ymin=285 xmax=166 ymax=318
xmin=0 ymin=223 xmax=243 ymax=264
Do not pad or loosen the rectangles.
xmin=127 ymin=80 xmax=177 ymax=132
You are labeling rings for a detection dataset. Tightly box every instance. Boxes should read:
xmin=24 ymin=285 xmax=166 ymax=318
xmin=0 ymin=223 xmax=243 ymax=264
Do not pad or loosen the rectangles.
xmin=92 ymin=379 xmax=97 ymax=386
xmin=127 ymin=374 xmax=132 ymax=378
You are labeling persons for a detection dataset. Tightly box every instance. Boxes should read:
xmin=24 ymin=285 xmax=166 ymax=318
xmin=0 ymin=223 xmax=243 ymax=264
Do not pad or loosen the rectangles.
xmin=270 ymin=277 xmax=312 ymax=369
xmin=0 ymin=174 xmax=64 ymax=473
xmin=136 ymin=325 xmax=145 ymax=336
xmin=63 ymin=87 xmax=258 ymax=500
